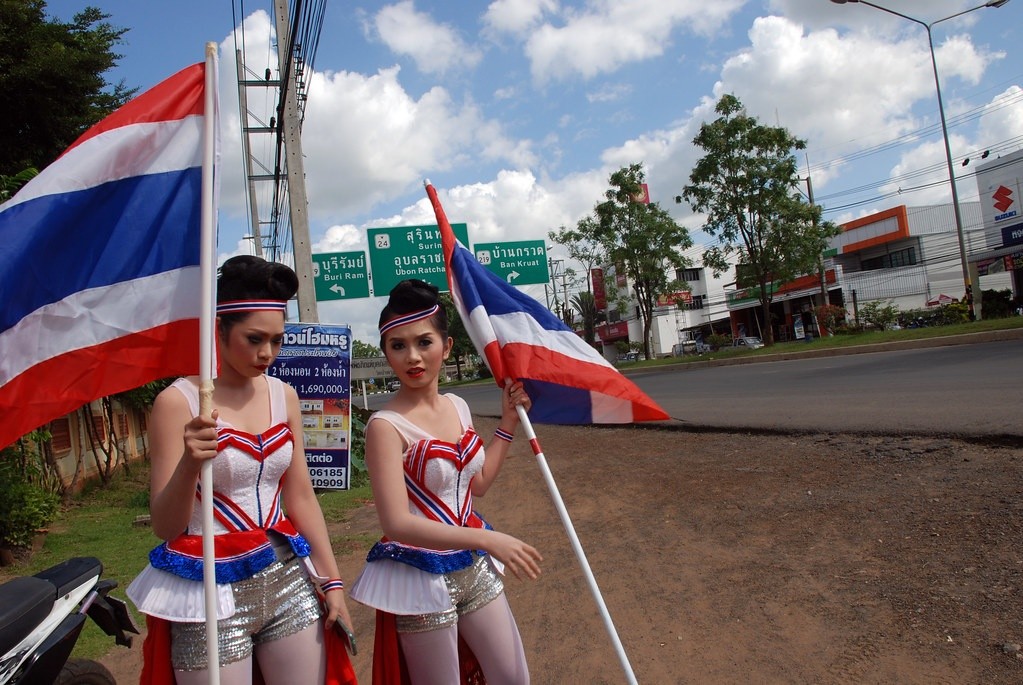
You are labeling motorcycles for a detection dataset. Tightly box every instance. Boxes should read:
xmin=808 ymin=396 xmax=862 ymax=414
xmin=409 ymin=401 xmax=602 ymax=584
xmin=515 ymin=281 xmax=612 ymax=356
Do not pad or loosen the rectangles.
xmin=0 ymin=556 xmax=140 ymax=685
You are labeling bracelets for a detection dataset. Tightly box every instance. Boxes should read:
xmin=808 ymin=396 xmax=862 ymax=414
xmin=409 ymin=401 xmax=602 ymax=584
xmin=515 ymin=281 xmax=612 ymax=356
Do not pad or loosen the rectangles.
xmin=320 ymin=579 xmax=343 ymax=594
xmin=494 ymin=428 xmax=514 ymax=442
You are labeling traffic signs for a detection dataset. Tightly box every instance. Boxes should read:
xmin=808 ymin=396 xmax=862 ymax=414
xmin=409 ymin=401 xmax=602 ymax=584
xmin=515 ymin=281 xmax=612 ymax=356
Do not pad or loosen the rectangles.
xmin=367 ymin=223 xmax=471 ymax=297
xmin=473 ymin=239 xmax=549 ymax=287
xmin=310 ymin=250 xmax=370 ymax=301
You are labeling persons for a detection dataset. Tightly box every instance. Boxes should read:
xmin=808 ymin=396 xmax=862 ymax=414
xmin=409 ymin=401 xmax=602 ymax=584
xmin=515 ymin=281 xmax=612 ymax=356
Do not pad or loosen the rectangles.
xmin=126 ymin=255 xmax=358 ymax=685
xmin=347 ymin=279 xmax=532 ymax=685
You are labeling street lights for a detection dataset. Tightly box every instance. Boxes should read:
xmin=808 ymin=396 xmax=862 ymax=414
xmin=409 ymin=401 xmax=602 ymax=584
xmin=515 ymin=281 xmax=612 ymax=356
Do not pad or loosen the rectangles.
xmin=832 ymin=0 xmax=1009 ymax=321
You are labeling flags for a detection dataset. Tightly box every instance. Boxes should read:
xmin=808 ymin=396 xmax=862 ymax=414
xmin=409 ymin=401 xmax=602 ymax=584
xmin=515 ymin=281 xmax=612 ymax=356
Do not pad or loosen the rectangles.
xmin=0 ymin=60 xmax=220 ymax=448
xmin=426 ymin=183 xmax=672 ymax=424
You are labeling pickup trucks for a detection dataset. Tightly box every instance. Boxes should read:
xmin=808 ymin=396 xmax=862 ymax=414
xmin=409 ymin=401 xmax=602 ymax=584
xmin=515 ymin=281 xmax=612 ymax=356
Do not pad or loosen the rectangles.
xmin=719 ymin=337 xmax=765 ymax=352
xmin=617 ymin=352 xmax=646 ymax=363
